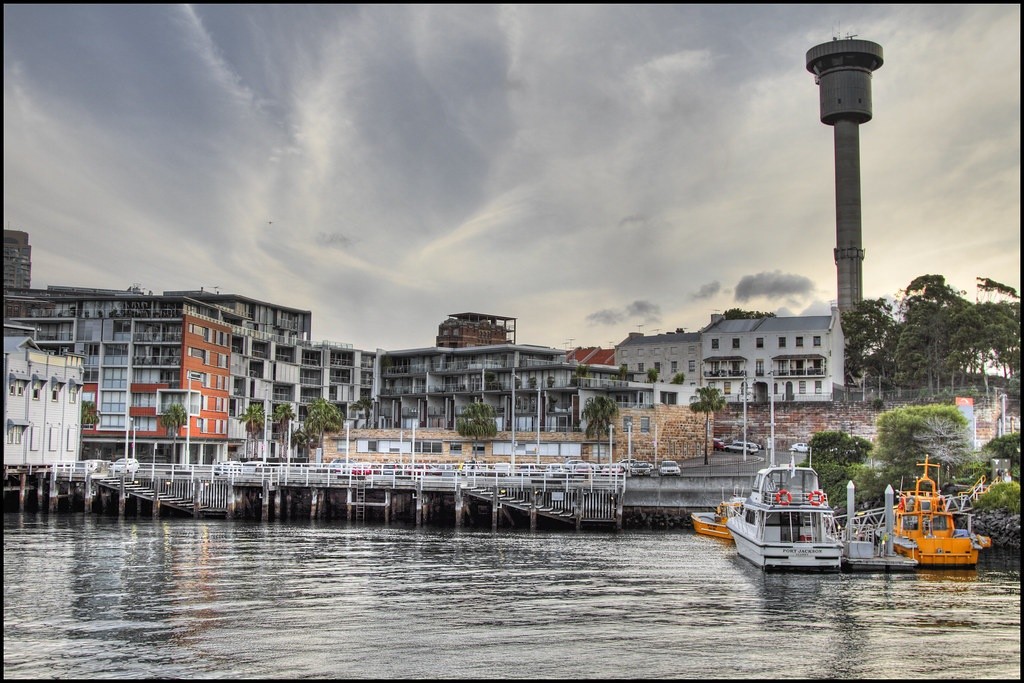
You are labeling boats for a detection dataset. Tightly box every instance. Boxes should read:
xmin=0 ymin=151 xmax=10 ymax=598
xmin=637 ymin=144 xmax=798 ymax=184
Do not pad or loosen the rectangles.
xmin=726 ymin=447 xmax=844 ymax=573
xmin=883 ymin=454 xmax=991 ymax=565
xmin=691 ymin=485 xmax=747 ymax=540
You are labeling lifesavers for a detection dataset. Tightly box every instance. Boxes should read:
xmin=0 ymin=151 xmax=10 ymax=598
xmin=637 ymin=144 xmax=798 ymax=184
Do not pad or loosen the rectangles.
xmin=808 ymin=490 xmax=824 ymax=506
xmin=898 ymin=498 xmax=905 ymax=510
xmin=776 ymin=490 xmax=792 ymax=506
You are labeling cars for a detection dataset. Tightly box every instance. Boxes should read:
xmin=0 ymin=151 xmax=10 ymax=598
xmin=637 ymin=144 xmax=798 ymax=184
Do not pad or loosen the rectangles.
xmin=713 ymin=437 xmax=809 ymax=455
xmin=214 ymin=460 xmax=682 ymax=477
xmin=110 ymin=458 xmax=140 ymax=474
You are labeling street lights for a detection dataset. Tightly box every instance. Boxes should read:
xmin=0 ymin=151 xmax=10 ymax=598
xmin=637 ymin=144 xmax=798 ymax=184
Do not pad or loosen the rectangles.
xmin=511 ymin=367 xmax=520 ymax=476
xmin=767 ymin=370 xmax=776 ymax=466
xmin=263 ymin=384 xmax=269 ymax=463
xmin=185 ymin=376 xmax=202 ymax=471
xmin=743 ymin=375 xmax=748 ymax=461
xmin=536 ymin=387 xmax=541 ymax=464
